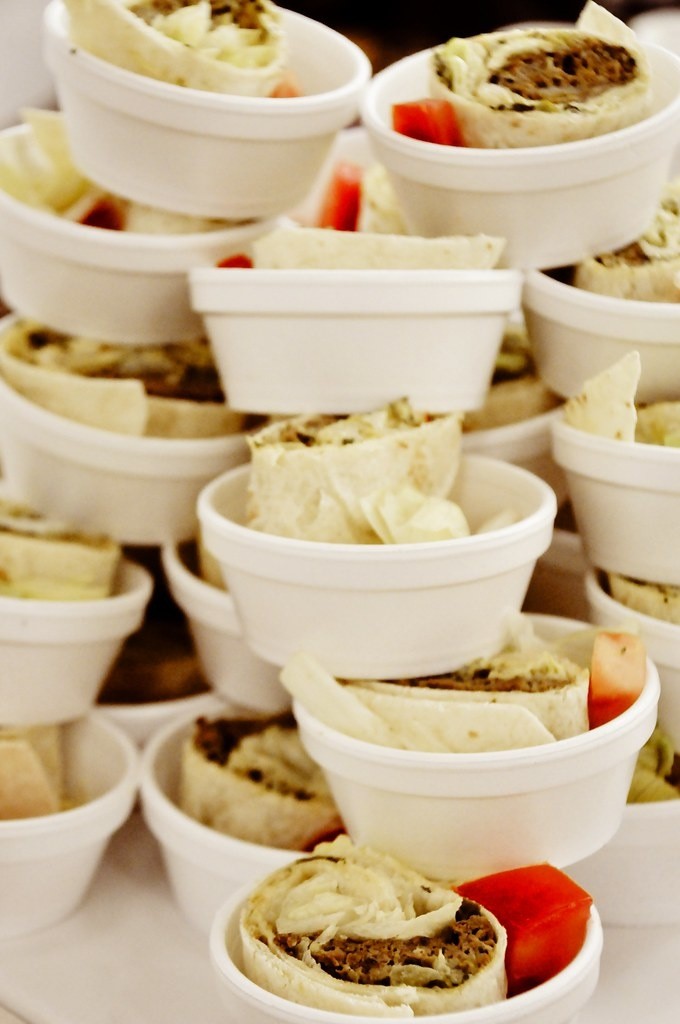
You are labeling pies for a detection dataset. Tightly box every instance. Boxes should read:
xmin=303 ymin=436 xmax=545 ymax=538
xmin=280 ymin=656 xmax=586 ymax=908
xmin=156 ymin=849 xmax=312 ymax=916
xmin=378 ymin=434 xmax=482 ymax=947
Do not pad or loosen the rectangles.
xmin=0 ymin=0 xmax=680 ymax=1024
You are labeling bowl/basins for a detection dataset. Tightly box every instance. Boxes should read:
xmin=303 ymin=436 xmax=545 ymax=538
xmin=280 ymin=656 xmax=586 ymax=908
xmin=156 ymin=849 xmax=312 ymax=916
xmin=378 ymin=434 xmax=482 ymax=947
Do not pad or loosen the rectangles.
xmin=0 ymin=0 xmax=680 ymax=1024
